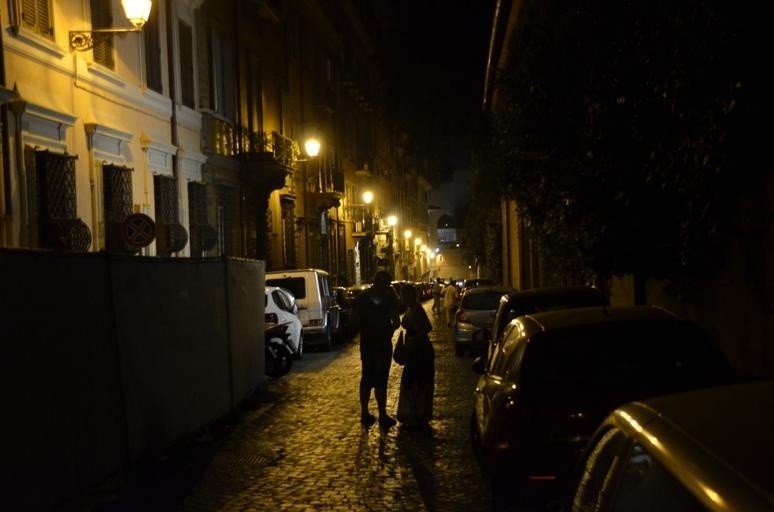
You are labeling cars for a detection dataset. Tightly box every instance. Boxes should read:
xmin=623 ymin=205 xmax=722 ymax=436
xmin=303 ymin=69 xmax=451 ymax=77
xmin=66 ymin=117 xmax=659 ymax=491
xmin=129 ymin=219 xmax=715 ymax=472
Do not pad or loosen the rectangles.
xmin=264 ymin=286 xmax=304 ymax=359
xmin=332 ymin=279 xmax=446 ymax=339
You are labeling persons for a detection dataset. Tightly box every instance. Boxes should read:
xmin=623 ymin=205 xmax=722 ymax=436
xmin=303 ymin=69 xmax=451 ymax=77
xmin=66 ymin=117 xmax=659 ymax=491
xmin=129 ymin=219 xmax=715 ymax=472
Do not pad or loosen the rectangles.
xmin=350 ymin=267 xmax=399 ymax=426
xmin=392 ymin=282 xmax=440 ymax=439
xmin=428 ymin=276 xmax=460 ymax=327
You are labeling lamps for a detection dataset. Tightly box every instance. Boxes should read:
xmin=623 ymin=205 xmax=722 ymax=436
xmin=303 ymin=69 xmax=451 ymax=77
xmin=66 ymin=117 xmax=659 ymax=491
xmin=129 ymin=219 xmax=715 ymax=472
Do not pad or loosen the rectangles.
xmin=64 ymin=1 xmax=153 ymax=52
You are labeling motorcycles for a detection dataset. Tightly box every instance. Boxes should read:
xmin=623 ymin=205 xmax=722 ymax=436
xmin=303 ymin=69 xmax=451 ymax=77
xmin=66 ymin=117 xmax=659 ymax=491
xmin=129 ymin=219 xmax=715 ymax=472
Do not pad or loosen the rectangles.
xmin=265 ymin=317 xmax=297 ymax=379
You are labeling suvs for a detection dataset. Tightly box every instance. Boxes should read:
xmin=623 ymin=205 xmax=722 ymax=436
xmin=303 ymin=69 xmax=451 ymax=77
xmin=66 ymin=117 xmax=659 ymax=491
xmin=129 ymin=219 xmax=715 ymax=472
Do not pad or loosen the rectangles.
xmin=265 ymin=269 xmax=342 ymax=350
